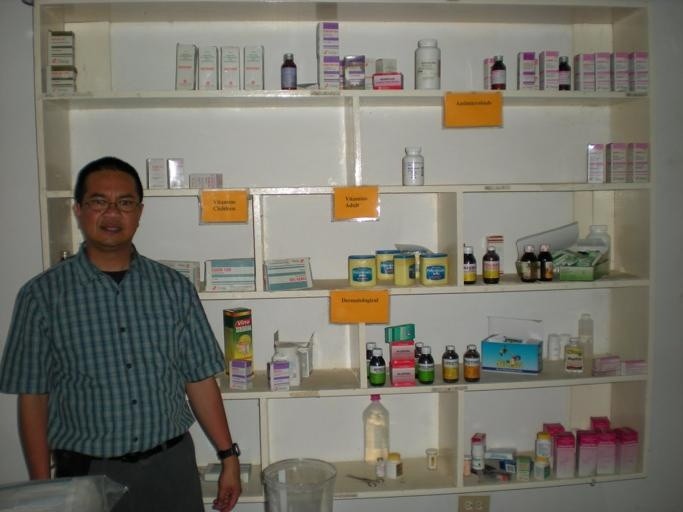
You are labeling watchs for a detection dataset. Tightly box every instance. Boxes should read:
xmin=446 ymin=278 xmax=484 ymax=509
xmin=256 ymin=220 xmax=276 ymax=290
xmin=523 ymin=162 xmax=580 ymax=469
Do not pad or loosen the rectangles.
xmin=218 ymin=443 xmax=240 ymax=458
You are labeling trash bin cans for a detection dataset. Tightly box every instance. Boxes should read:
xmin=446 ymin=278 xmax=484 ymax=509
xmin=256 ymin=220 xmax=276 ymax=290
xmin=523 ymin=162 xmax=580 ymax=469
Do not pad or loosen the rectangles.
xmin=0 ymin=475 xmax=106 ymax=512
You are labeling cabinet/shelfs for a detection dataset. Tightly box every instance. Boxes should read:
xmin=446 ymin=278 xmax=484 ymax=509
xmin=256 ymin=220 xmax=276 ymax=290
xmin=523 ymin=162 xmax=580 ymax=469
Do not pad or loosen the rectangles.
xmin=32 ymin=1 xmax=656 ymax=505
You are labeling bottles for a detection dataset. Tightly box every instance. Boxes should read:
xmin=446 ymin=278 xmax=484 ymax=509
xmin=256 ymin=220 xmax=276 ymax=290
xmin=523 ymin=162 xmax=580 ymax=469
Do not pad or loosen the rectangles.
xmin=464 ymin=437 xmax=485 ymax=477
xmin=415 ymin=342 xmax=482 ymax=385
xmin=534 ymin=432 xmax=554 ymax=481
xmin=522 ymin=246 xmax=555 ymax=281
xmin=427 ymin=449 xmax=439 ymax=470
xmin=545 ymin=313 xmax=594 ymax=373
xmin=278 ymin=52 xmax=298 ymax=90
xmin=462 ymin=247 xmax=478 ymax=285
xmin=366 ymin=341 xmax=386 ymax=387
xmin=557 ymin=57 xmax=572 ymax=91
xmin=402 ymin=145 xmax=425 ymax=186
xmin=414 ymin=40 xmax=441 ymax=90
xmin=363 ymin=393 xmax=389 ymax=462
xmin=377 ymin=453 xmax=404 ymax=479
xmin=482 ymin=246 xmax=501 ymax=286
xmin=489 ymin=55 xmax=507 ymax=90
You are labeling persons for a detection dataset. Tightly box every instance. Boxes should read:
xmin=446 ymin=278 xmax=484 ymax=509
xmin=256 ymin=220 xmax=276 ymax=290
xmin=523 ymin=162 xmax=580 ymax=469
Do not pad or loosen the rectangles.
xmin=1 ymin=156 xmax=242 ymax=512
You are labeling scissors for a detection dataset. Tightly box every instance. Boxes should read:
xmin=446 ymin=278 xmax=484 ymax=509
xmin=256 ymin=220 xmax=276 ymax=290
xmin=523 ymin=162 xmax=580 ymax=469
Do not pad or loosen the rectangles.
xmin=345 ymin=474 xmax=385 ymax=488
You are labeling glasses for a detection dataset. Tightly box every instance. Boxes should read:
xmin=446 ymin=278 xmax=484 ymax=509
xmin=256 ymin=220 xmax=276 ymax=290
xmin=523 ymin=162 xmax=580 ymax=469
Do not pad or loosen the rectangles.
xmin=78 ymin=199 xmax=141 ymax=213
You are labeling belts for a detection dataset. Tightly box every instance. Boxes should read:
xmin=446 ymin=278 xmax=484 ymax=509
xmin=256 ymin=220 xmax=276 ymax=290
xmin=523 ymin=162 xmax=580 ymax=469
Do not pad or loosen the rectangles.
xmin=52 ymin=430 xmax=189 ymax=466
